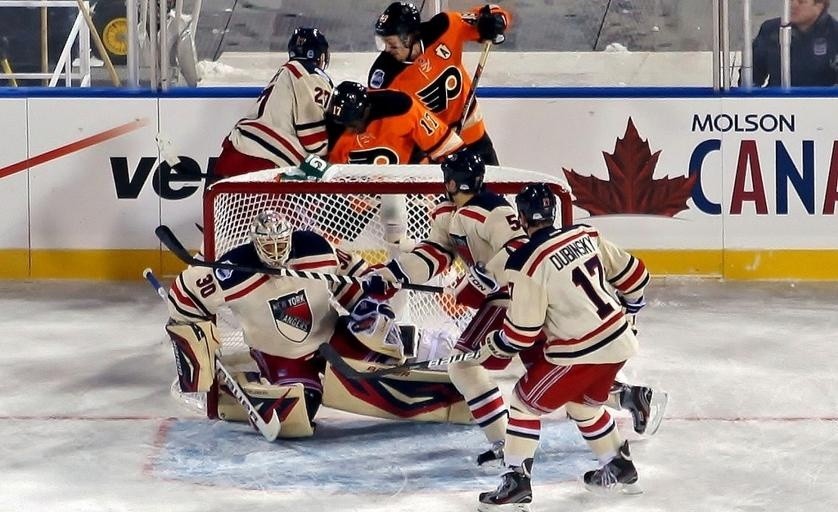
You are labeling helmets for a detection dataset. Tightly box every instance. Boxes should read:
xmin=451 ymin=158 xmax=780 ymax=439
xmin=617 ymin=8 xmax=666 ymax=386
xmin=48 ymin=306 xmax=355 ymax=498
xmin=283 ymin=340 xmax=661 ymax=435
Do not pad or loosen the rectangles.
xmin=441 ymin=150 xmax=486 ymax=196
xmin=516 ymin=180 xmax=557 ymax=227
xmin=329 ymin=81 xmax=369 ymax=125
xmin=249 ymin=209 xmax=293 ymax=267
xmin=288 ymin=25 xmax=330 ymax=70
xmin=375 ymin=2 xmax=423 ymax=53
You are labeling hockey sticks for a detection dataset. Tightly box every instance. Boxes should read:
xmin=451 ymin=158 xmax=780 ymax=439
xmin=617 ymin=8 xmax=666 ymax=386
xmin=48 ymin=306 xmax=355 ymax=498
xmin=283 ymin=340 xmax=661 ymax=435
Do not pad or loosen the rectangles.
xmin=155 ymin=225 xmax=453 ymax=293
xmin=155 ymin=132 xmax=230 ymax=180
xmin=142 ymin=268 xmax=281 ymax=442
xmin=318 ymin=343 xmax=491 ymax=379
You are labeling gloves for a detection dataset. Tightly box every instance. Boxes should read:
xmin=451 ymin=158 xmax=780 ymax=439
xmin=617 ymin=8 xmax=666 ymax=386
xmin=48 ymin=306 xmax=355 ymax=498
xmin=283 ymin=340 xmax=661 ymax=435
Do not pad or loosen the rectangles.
xmin=448 ymin=263 xmax=497 ymax=308
xmin=478 ymin=13 xmax=505 ymax=44
xmin=484 ymin=329 xmax=520 ymax=361
xmin=358 ymin=259 xmax=407 ymax=303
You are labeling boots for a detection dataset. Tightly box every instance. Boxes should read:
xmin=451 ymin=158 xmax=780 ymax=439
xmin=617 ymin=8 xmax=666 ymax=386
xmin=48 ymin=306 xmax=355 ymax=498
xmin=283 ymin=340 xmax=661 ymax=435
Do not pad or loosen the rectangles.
xmin=477 ymin=440 xmax=505 ymax=464
xmin=584 ymin=439 xmax=639 ymax=485
xmin=611 ymin=381 xmax=653 ymax=434
xmin=480 ymin=457 xmax=534 ymax=505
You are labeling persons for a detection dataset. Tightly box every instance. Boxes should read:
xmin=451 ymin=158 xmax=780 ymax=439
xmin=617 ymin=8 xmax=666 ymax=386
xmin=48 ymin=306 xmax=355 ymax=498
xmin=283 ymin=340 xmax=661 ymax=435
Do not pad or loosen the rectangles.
xmin=357 ymin=148 xmax=653 ymax=465
xmin=477 ymin=179 xmax=650 ymax=507
xmin=366 ymin=1 xmax=510 ymax=168
xmin=164 ymin=208 xmax=481 ymax=442
xmin=733 ymin=0 xmax=837 ymax=89
xmin=191 ymin=26 xmax=334 ymax=266
xmin=326 ymin=77 xmax=473 ymax=328
